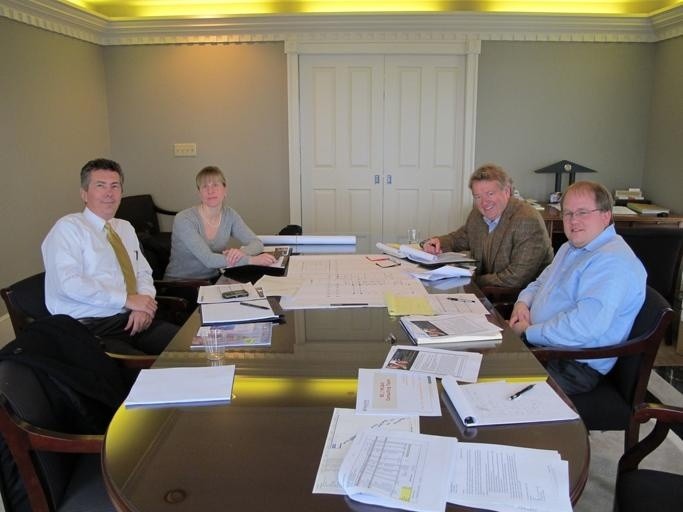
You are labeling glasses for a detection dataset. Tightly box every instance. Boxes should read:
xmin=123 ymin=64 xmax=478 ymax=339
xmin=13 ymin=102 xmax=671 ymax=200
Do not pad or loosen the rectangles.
xmin=559 ymin=209 xmax=602 ymax=220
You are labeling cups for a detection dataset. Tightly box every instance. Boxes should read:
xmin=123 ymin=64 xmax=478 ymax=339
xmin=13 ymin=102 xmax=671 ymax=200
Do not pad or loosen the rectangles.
xmin=199 ymin=328 xmax=226 ymax=360
xmin=406 ymin=229 xmax=422 ymax=247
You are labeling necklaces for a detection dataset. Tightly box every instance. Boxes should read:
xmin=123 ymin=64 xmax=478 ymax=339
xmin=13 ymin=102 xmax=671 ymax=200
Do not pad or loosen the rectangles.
xmin=199 ymin=205 xmax=222 ymax=228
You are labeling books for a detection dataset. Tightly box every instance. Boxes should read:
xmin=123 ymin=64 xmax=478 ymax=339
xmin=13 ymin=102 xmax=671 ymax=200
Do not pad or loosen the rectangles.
xmin=442 ymin=375 xmax=580 ymax=424
xmin=126 ymin=364 xmax=238 ymax=405
xmin=626 ymin=201 xmax=671 ymax=216
xmin=376 ymin=240 xmax=421 ymax=259
xmin=399 ymin=309 xmax=505 ymax=346
xmin=409 ymin=264 xmax=473 ymax=282
xmin=400 ymin=243 xmax=476 ymax=264
xmin=193 ymin=282 xmax=278 ymax=349
xmin=524 ymin=198 xmax=544 ymax=211
xmin=611 ymin=205 xmax=631 ymax=215
xmin=613 ymin=188 xmax=645 ymax=201
xmin=354 ymin=365 xmax=443 ymax=418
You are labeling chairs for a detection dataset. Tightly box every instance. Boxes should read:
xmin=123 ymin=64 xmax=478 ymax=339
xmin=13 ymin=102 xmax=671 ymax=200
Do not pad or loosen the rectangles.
xmin=487 ymin=256 xmax=539 ymax=324
xmin=609 ymin=395 xmax=682 ymax=508
xmin=0 ymin=270 xmax=187 ymax=373
xmin=0 ymin=337 xmax=120 ymax=512
xmin=513 ymin=283 xmax=674 ymax=472
xmin=113 ymin=194 xmax=219 ymax=311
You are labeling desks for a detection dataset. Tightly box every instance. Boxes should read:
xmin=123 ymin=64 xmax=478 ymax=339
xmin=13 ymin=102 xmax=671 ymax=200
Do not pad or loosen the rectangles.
xmin=98 ymin=237 xmax=594 ymax=510
xmin=522 ymin=195 xmax=682 ymax=344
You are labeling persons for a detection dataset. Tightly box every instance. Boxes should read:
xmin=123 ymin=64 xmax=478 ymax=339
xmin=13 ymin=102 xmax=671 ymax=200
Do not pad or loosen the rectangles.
xmin=39 ymin=158 xmax=188 ymax=376
xmin=503 ymin=178 xmax=651 ymax=403
xmin=160 ymin=166 xmax=277 ymax=289
xmin=420 ymin=162 xmax=556 ymax=301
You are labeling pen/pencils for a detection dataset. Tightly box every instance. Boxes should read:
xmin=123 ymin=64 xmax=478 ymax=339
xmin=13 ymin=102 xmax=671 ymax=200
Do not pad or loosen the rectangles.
xmin=509 ymin=384 xmax=536 ymax=401
xmin=330 ymin=303 xmax=368 ymax=305
xmin=240 ymin=303 xmax=270 ymax=309
xmin=446 ymin=298 xmax=476 ymax=303
xmin=431 ymin=243 xmax=436 ymax=247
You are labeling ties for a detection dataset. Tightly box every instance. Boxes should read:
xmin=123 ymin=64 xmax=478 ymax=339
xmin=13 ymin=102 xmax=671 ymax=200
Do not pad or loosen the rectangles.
xmin=104 ymin=223 xmax=136 ymax=294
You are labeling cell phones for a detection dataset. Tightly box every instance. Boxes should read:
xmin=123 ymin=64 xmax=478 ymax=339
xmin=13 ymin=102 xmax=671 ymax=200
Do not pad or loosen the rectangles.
xmin=222 ymin=290 xmax=249 ymax=298
